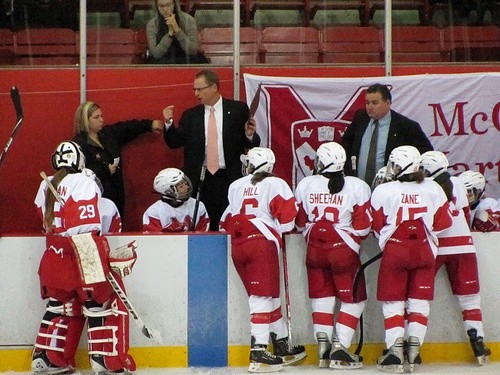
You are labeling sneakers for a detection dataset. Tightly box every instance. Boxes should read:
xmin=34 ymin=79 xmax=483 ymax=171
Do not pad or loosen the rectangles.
xmin=328 ymin=335 xmax=363 ymax=369
xmin=467 ymin=329 xmax=492 ymax=366
xmin=270 ymin=332 xmax=307 ymax=366
xmin=247 ymin=336 xmax=284 ymax=372
xmin=316 ymin=332 xmax=333 ymax=368
xmin=405 ymin=336 xmax=423 ymax=373
xmin=376 ymin=337 xmax=405 ymax=373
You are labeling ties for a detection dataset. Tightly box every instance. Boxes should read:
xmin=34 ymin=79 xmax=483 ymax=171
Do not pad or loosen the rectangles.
xmin=365 ymin=120 xmax=379 ymax=187
xmin=207 ymin=107 xmax=219 ymax=175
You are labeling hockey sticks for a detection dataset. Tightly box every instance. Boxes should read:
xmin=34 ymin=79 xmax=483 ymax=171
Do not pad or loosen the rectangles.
xmin=282 ymin=232 xmax=300 ymax=351
xmin=192 ymin=163 xmax=208 ymax=232
xmin=40 ymin=171 xmax=154 ymax=338
xmin=352 ymin=251 xmax=384 ymax=303
xmin=0 ymin=84 xmax=25 ymax=163
xmin=351 ymin=155 xmax=364 ymax=353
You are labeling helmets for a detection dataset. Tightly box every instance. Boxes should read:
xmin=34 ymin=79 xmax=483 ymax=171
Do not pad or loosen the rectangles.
xmin=54 ymin=140 xmax=85 ymax=173
xmin=459 ymin=170 xmax=486 ymax=206
xmin=372 ymin=166 xmax=388 ymax=190
xmin=386 ymin=145 xmax=425 ymax=181
xmin=153 ymin=168 xmax=193 ymax=201
xmin=314 ymin=142 xmax=346 ymax=173
xmin=421 ymin=151 xmax=449 ymax=180
xmin=83 ymin=168 xmax=104 ymax=195
xmin=240 ymin=147 xmax=276 ymax=175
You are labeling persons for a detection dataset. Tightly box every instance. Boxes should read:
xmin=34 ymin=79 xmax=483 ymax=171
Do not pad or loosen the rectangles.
xmin=295 ymin=142 xmax=372 ymax=367
xmin=146 ymin=0 xmax=209 ymax=64
xmin=81 ymin=168 xmax=121 ymax=233
xmin=369 ymin=146 xmax=452 ymax=372
xmin=163 ymin=70 xmax=261 ymax=231
xmin=342 ymin=84 xmax=434 ymax=188
xmin=372 ymin=166 xmax=392 ymax=192
xmin=143 ymin=167 xmax=210 ymax=232
xmin=384 ymin=151 xmax=491 ymax=368
xmin=31 ymin=140 xmax=136 ymax=375
xmin=227 ymin=147 xmax=307 ymax=372
xmin=459 ymin=170 xmax=500 ymax=231
xmin=71 ymin=102 xmax=164 ymax=235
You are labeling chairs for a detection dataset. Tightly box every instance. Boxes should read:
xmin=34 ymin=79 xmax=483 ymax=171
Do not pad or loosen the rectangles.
xmin=0 ymin=0 xmax=500 ymax=68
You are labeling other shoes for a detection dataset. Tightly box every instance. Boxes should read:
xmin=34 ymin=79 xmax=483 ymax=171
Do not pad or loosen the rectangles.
xmin=90 ymin=359 xmax=126 ymax=375
xmin=33 ymin=353 xmax=71 ymax=374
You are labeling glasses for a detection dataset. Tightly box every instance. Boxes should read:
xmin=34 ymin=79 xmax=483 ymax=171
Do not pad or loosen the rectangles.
xmin=157 ymin=1 xmax=174 ymax=9
xmin=192 ymin=84 xmax=213 ymax=92
